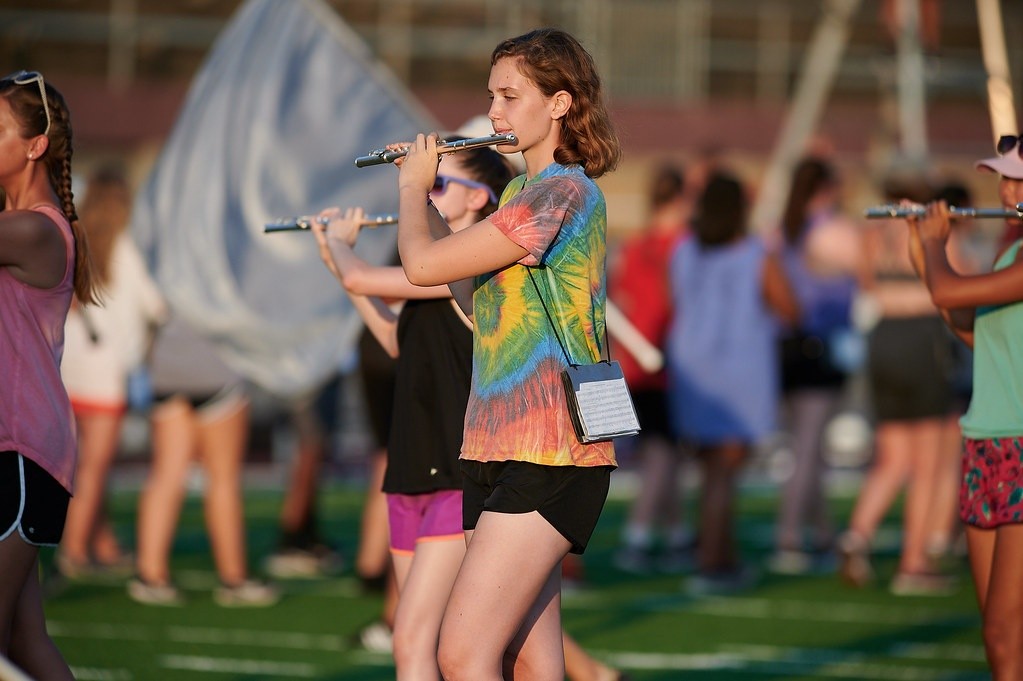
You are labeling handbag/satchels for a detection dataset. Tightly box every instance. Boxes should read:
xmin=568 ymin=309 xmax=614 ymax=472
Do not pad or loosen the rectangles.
xmin=562 ymin=359 xmax=641 ymax=444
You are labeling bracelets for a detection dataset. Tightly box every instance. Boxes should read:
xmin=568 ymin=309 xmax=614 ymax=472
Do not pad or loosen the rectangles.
xmin=427 ymin=199 xmax=432 ymax=206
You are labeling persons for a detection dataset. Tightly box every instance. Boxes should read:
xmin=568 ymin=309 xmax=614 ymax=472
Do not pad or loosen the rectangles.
xmin=608 ymin=156 xmax=974 ymax=598
xmin=901 ymin=136 xmax=1023 ymax=681
xmin=310 ymin=134 xmax=517 ymax=681
xmin=57 ymin=174 xmax=397 ymax=608
xmin=385 ymin=29 xmax=623 ymax=681
xmin=0 ymin=71 xmax=99 ymax=681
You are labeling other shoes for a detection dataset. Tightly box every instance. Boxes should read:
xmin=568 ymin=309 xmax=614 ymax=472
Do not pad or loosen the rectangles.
xmin=129 ymin=578 xmax=179 ymax=605
xmin=215 ymin=580 xmax=277 ymax=608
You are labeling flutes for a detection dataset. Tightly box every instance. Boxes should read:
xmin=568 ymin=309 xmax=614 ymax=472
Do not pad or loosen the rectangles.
xmin=264 ymin=214 xmax=399 ymax=234
xmin=354 ymin=131 xmax=518 ymax=169
xmin=862 ymin=201 xmax=1023 ymax=221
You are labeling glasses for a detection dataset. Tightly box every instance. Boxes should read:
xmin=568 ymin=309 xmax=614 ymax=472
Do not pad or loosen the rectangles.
xmin=429 ymin=174 xmax=498 ymax=206
xmin=996 ymin=134 xmax=1023 ymax=158
xmin=3 ymin=70 xmax=50 ymax=136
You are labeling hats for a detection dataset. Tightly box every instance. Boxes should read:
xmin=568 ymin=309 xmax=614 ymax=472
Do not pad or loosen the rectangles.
xmin=975 ymin=141 xmax=1023 ymax=181
